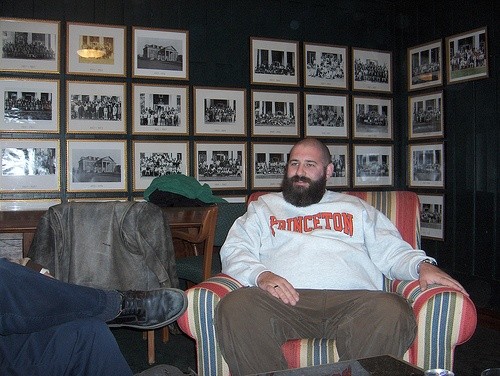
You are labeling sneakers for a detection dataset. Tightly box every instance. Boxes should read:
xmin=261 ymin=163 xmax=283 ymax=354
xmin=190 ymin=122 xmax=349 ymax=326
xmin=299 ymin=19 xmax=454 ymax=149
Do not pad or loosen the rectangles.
xmin=105 ymin=287 xmax=188 ymax=329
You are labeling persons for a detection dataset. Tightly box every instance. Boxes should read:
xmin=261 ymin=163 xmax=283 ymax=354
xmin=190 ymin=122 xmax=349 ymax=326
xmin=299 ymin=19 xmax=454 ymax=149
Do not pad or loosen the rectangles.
xmin=140 ymin=152 xmax=182 ymax=177
xmin=199 ymin=159 xmax=242 ymax=179
xmin=414 ymin=109 xmax=440 ymax=122
xmin=415 ymin=160 xmax=439 ymax=172
xmin=253 ymin=111 xmax=294 ymax=128
xmin=203 ymin=106 xmax=235 ymax=122
xmin=255 ymin=160 xmax=286 ymax=177
xmin=420 ymin=209 xmax=440 ymax=223
xmin=0 ymin=257 xmax=189 ymax=376
xmin=2 ymin=40 xmax=54 ymax=59
xmin=214 ymin=138 xmax=469 ymax=376
xmin=304 ymin=57 xmax=388 ymax=176
xmin=255 ymin=63 xmax=294 ymax=75
xmin=451 ymin=45 xmax=484 ymax=70
xmin=141 ymin=105 xmax=179 ymax=126
xmin=71 ymin=97 xmax=120 ymax=120
xmin=412 ymin=61 xmax=440 ymax=76
xmin=4 ymin=97 xmax=51 ymax=111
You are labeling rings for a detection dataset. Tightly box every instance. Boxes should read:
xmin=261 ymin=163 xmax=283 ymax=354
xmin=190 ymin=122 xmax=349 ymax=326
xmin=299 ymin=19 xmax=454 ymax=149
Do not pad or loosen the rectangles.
xmin=273 ymin=285 xmax=279 ymax=289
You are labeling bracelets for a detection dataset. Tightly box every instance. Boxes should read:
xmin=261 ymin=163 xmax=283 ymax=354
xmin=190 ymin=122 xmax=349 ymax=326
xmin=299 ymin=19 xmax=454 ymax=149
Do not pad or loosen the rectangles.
xmin=416 ymin=259 xmax=436 ymax=273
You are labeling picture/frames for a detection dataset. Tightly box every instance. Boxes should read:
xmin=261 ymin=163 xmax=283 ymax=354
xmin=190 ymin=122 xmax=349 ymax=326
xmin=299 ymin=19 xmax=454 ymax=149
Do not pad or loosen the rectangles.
xmin=193 ymin=85 xmax=249 ymax=191
xmin=406 ymin=143 xmax=445 ymax=189
xmin=64 ymin=21 xmax=128 ymax=194
xmin=303 ymin=43 xmax=349 ymax=189
xmin=351 ymin=46 xmax=393 ymax=94
xmin=353 ymin=96 xmax=393 ymax=140
xmin=409 ymin=91 xmax=444 ymax=139
xmin=408 ymin=40 xmax=444 ymax=91
xmin=0 ymin=18 xmax=62 ymax=194
xmin=446 ymin=27 xmax=488 ymax=84
xmin=353 ymin=144 xmax=394 ymax=189
xmin=416 ymin=192 xmax=444 ymax=241
xmin=249 ymin=36 xmax=300 ymax=189
xmin=131 ymin=25 xmax=190 ymax=193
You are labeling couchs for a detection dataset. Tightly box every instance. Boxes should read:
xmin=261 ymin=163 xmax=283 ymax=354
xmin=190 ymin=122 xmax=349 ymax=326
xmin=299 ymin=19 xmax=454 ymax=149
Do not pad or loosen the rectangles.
xmin=176 ymin=191 xmax=476 ymax=376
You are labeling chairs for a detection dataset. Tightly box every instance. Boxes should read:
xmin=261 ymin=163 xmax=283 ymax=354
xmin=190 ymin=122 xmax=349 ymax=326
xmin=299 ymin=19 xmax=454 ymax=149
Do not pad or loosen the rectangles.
xmin=0 ymin=204 xmax=248 ymax=366
